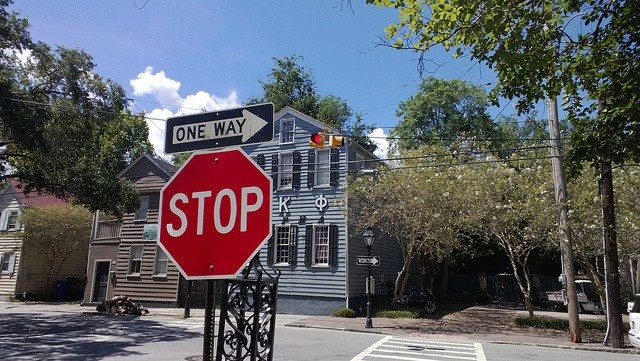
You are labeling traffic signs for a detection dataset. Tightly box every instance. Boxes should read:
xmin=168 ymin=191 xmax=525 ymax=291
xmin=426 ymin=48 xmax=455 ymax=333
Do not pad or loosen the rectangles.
xmin=356 ymin=255 xmax=383 ymax=267
xmin=161 ymin=101 xmax=275 ymax=159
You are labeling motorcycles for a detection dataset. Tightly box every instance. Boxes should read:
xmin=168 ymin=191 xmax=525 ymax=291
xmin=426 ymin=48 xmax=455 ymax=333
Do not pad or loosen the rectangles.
xmin=396 ymin=285 xmax=438 ymax=313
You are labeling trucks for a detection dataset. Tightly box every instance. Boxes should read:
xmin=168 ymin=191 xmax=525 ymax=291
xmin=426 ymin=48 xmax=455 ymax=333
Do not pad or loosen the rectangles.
xmin=536 ymin=278 xmax=606 ymax=319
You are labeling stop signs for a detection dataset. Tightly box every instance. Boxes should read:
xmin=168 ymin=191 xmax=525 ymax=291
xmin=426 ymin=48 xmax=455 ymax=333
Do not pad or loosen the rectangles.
xmin=158 ymin=143 xmax=271 ymax=281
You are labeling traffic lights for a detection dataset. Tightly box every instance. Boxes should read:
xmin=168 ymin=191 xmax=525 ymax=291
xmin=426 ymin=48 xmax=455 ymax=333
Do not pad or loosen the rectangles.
xmin=330 ymin=135 xmax=343 ymax=150
xmin=309 ymin=131 xmax=326 ymax=148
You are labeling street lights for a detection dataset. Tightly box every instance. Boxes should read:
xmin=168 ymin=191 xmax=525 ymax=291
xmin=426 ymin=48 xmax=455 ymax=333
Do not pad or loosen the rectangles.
xmin=363 ymin=229 xmax=378 ymax=328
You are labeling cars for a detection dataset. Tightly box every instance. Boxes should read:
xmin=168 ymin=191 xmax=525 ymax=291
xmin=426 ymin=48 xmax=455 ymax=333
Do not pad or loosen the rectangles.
xmin=629 ymin=295 xmax=640 ymax=349
